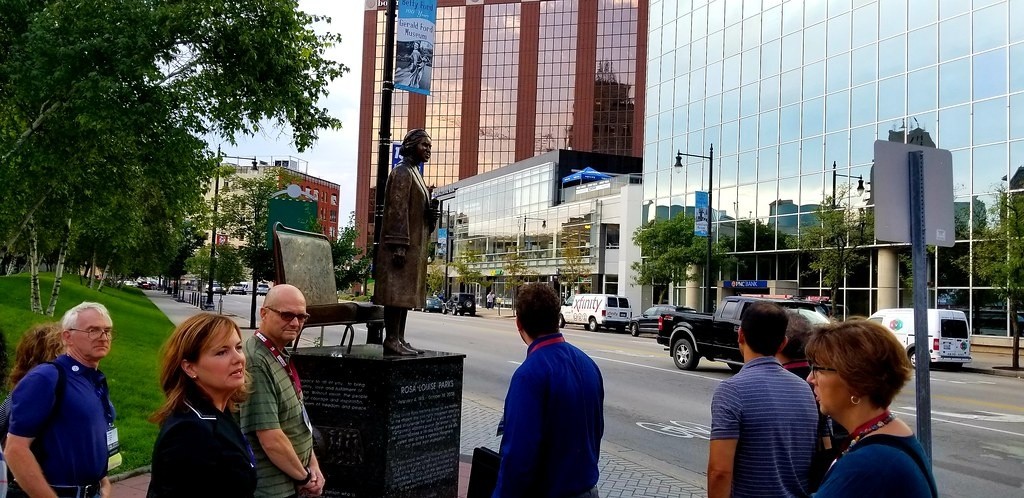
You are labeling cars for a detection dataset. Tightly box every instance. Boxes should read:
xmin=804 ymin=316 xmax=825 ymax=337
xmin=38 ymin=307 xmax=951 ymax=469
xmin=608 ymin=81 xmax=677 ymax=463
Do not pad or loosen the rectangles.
xmin=139 ymin=277 xmax=271 ymax=294
xmin=425 ymin=296 xmax=443 ymax=313
xmin=628 ymin=305 xmax=697 ymax=338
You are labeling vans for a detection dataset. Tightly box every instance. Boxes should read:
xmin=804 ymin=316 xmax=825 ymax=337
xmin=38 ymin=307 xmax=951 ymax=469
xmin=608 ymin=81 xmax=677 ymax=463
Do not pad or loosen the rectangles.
xmin=559 ymin=293 xmax=633 ymax=332
xmin=863 ymin=307 xmax=972 ymax=372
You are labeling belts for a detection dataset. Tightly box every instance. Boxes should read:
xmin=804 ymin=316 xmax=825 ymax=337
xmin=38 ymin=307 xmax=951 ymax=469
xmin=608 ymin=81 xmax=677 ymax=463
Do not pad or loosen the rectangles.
xmin=9 ymin=480 xmax=101 ymax=498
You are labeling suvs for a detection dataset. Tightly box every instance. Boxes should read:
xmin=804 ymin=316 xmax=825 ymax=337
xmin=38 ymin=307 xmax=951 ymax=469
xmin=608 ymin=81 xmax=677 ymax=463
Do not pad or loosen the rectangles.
xmin=441 ymin=292 xmax=477 ymax=316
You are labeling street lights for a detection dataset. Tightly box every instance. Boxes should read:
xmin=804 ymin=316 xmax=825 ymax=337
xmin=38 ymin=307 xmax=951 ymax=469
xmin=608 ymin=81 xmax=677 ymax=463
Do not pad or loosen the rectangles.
xmin=831 ymin=162 xmax=865 ymax=209
xmin=206 ymin=144 xmax=259 ymax=309
xmin=523 ymin=215 xmax=546 ymax=250
xmin=674 ymin=143 xmax=714 ymax=312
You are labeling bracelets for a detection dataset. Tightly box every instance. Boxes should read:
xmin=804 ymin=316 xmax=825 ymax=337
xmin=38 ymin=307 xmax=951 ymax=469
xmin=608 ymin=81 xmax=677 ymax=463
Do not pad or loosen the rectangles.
xmin=299 ymin=467 xmax=311 ymax=486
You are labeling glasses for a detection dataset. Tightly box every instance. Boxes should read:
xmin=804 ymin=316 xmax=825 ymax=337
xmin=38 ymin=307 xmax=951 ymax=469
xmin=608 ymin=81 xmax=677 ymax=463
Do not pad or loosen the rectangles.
xmin=807 ymin=361 xmax=838 ymax=379
xmin=66 ymin=326 xmax=118 ymax=341
xmin=264 ymin=306 xmax=310 ymax=323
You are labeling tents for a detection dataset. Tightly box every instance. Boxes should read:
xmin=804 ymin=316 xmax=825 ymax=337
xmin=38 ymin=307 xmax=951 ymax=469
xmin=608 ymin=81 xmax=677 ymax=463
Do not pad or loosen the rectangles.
xmin=561 ymin=167 xmax=613 ymax=202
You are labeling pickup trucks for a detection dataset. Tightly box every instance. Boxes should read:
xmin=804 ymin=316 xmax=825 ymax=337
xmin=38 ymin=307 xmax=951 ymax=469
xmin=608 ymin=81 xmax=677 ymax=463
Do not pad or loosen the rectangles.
xmin=655 ymin=296 xmax=836 ymax=372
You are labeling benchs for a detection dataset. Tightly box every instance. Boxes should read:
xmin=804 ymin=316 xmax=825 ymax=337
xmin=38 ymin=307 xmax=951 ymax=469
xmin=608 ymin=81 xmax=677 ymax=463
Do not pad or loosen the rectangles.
xmin=272 ymin=221 xmax=386 ymax=353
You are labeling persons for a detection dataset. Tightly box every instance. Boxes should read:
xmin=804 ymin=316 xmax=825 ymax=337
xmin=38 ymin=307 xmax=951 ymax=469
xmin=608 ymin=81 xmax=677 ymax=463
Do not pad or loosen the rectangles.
xmin=239 ymin=284 xmax=326 ymax=498
xmin=805 ymin=319 xmax=939 ymax=498
xmin=0 ymin=302 xmax=115 ymax=498
xmin=146 ymin=314 xmax=257 ymax=498
xmin=433 ymin=293 xmax=444 ymax=299
xmin=491 ymin=283 xmax=604 ymax=498
xmin=487 ymin=290 xmax=495 ymax=310
xmin=708 ymin=301 xmax=852 ymax=498
xmin=374 ymin=129 xmax=441 ymax=356
